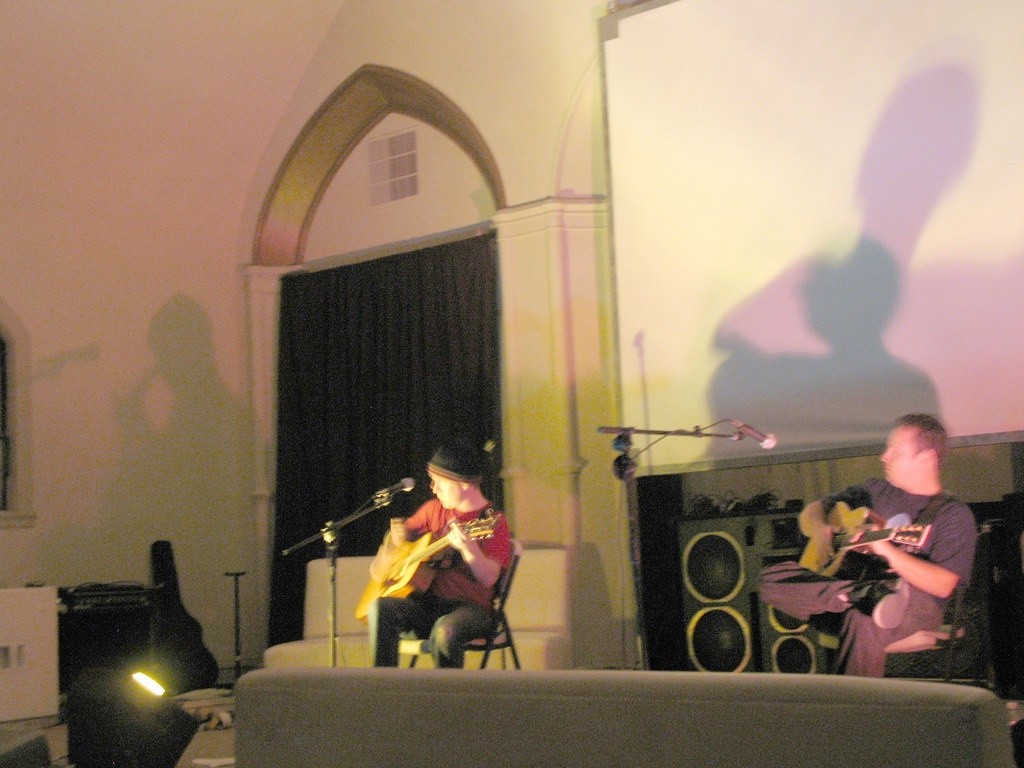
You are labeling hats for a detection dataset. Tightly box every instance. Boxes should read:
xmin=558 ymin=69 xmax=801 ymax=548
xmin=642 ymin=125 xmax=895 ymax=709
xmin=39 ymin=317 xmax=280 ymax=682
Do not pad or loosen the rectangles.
xmin=427 ymin=443 xmax=484 ymax=483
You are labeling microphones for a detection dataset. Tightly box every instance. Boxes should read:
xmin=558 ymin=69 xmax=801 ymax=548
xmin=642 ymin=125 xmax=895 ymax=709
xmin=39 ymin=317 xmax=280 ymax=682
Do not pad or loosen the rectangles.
xmin=729 ymin=418 xmax=778 ymax=450
xmin=373 ymin=477 xmax=415 ymax=500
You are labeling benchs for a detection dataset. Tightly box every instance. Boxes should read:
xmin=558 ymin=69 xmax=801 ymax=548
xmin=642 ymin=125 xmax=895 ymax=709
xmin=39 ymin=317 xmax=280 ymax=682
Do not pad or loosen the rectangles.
xmin=241 ymin=666 xmax=1012 ymax=768
xmin=262 ymin=549 xmax=573 ymax=669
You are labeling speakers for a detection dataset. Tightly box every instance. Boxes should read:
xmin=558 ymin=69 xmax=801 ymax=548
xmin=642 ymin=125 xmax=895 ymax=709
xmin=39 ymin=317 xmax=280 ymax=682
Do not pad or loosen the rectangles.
xmin=882 ymin=518 xmax=1024 ymax=701
xmin=56 ymin=583 xmax=193 ymax=768
xmin=677 ymin=510 xmax=846 ymax=676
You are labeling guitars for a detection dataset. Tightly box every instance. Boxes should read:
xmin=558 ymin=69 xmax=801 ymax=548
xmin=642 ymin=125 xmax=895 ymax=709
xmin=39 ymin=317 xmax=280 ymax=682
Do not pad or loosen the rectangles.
xmin=352 ymin=514 xmax=501 ymax=632
xmin=798 ymin=499 xmax=938 ymax=584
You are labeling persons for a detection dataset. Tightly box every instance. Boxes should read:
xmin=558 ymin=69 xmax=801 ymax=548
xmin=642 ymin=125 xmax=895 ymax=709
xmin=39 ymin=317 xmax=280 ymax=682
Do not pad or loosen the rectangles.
xmin=370 ymin=438 xmax=510 ymax=670
xmin=755 ymin=413 xmax=977 ymax=679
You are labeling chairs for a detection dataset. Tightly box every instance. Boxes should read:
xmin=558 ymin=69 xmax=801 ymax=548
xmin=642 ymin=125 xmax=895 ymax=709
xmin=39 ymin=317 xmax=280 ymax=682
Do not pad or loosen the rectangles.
xmin=398 ymin=554 xmax=521 ymax=668
xmin=884 ymin=519 xmax=998 ymax=692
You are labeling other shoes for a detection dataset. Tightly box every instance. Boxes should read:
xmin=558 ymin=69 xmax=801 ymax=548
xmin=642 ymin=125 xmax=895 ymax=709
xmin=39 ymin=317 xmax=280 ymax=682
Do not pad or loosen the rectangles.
xmin=849 ymin=577 xmax=910 ymax=628
